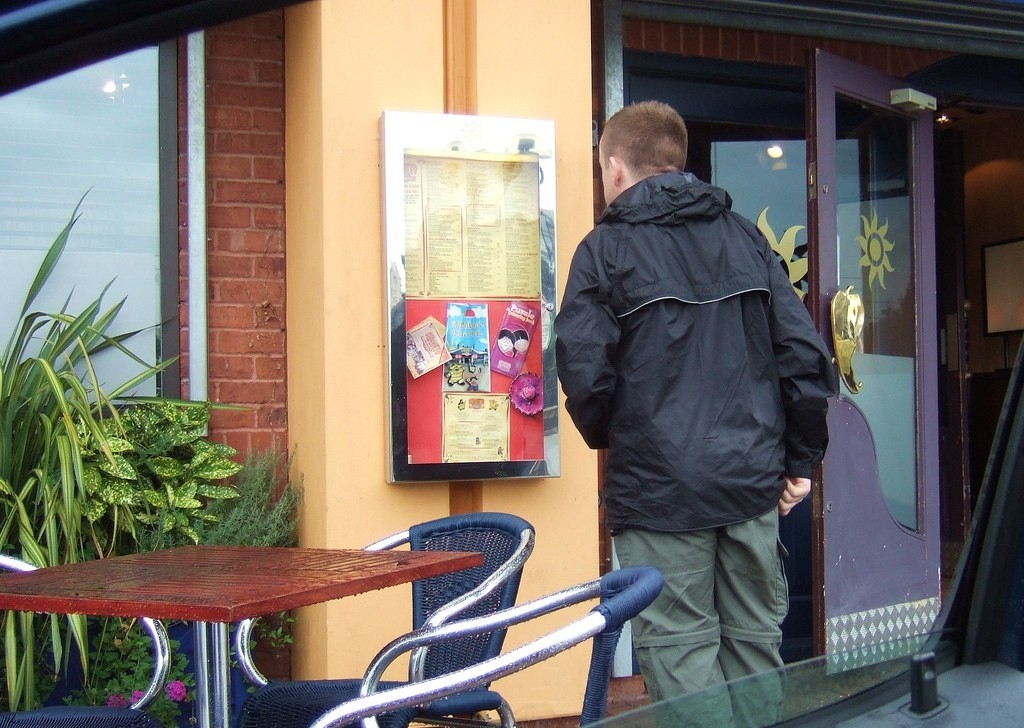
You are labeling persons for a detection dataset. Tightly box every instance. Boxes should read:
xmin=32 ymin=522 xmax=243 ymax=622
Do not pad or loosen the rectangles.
xmin=554 ymin=99 xmax=838 ymax=728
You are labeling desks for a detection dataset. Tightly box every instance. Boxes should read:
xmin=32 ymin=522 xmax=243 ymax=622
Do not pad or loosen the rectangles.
xmin=0 ymin=546 xmax=484 ymax=728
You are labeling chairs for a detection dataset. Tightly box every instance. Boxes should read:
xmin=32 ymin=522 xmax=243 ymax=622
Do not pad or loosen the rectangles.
xmin=0 ymin=555 xmax=171 ymax=728
xmin=233 ymin=506 xmax=535 ymax=728
xmin=311 ymin=567 xmax=664 ymax=728
